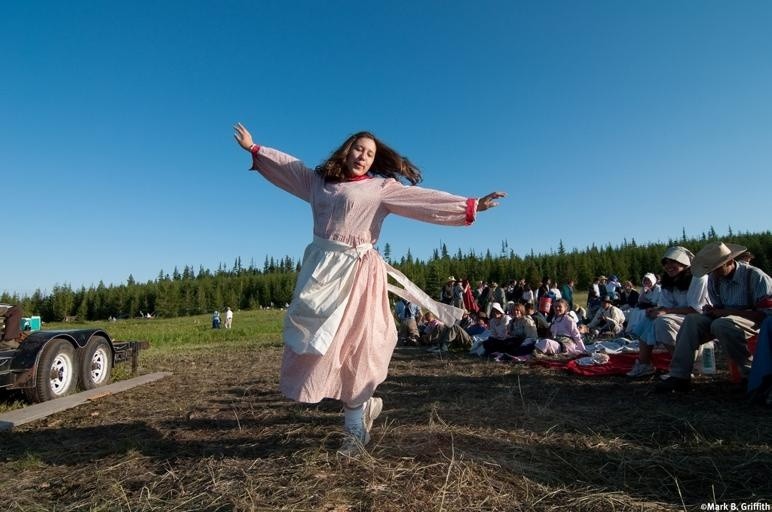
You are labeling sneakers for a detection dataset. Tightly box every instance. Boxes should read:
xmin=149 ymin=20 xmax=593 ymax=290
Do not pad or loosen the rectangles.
xmin=342 ymin=396 xmax=383 ymax=450
xmin=627 ymin=359 xmax=656 ymax=376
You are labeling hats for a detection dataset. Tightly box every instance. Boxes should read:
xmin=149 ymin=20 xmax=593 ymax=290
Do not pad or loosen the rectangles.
xmin=600 ymin=296 xmax=612 ymax=301
xmin=664 ymin=246 xmax=695 ymax=272
xmin=600 ymin=276 xmax=607 ymax=279
xmin=448 ymin=276 xmax=456 ymax=282
xmin=691 ymin=242 xmax=747 ymax=278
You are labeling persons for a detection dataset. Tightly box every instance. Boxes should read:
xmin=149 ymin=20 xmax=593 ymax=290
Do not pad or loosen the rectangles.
xmin=394 ymin=240 xmax=772 ymax=404
xmin=231 ymin=120 xmax=508 ymax=448
xmin=212 ymin=306 xmax=233 ymax=329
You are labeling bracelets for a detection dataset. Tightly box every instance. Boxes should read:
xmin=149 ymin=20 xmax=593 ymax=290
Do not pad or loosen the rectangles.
xmin=249 ymin=144 xmax=256 ymax=152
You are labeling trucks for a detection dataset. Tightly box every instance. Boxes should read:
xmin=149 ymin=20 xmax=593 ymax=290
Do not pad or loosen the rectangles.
xmin=1 ymin=301 xmax=149 ymax=401
